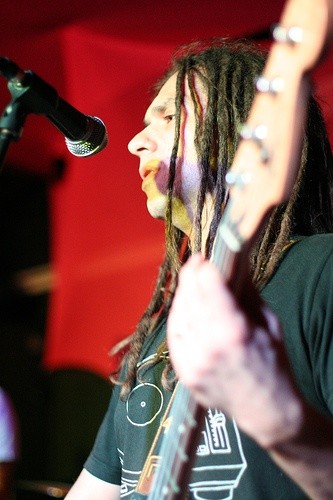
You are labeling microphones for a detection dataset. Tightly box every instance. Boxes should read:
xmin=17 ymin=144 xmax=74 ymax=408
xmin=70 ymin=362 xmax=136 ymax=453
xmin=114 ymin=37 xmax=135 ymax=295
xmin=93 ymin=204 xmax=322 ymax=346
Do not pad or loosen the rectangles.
xmin=0 ymin=54 xmax=108 ymax=157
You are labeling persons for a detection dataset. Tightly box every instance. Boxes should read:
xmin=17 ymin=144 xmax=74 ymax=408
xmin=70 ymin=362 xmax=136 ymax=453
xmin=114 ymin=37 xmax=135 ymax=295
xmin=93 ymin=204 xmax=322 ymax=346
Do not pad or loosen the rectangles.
xmin=0 ymin=387 xmax=18 ymax=500
xmin=64 ymin=44 xmax=333 ymax=500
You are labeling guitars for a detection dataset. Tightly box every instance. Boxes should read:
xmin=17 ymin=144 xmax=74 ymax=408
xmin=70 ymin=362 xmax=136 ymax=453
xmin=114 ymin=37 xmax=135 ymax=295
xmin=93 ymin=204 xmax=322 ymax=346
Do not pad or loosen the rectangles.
xmin=143 ymin=0 xmax=333 ymax=500
